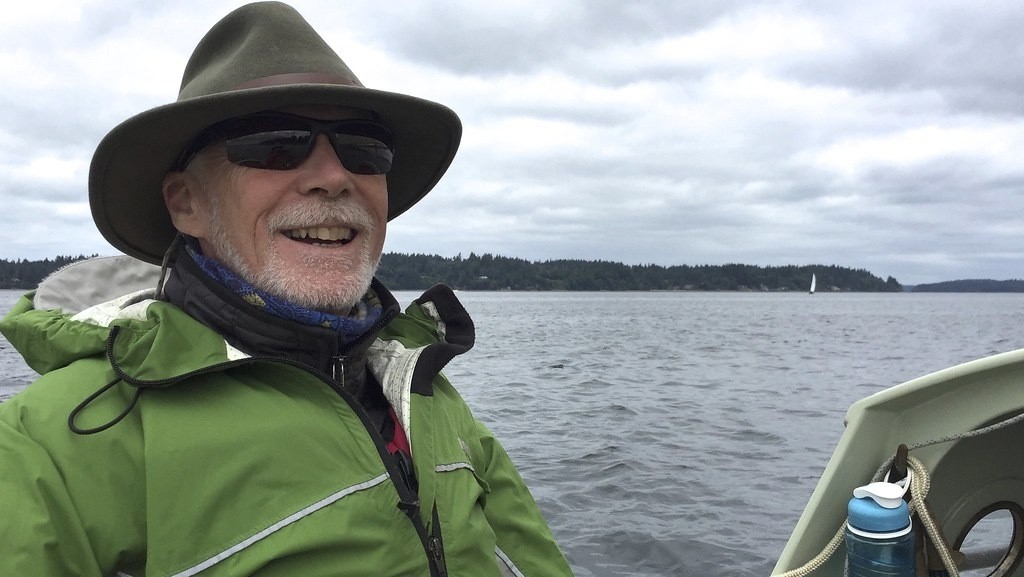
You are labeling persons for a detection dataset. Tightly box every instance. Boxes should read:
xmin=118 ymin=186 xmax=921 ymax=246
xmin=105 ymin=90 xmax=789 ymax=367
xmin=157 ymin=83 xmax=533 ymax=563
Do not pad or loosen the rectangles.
xmin=0 ymin=3 xmax=575 ymax=576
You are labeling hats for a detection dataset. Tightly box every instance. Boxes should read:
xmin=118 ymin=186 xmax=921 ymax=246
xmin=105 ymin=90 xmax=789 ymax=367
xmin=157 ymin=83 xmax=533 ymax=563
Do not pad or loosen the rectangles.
xmin=89 ymin=1 xmax=463 ymax=265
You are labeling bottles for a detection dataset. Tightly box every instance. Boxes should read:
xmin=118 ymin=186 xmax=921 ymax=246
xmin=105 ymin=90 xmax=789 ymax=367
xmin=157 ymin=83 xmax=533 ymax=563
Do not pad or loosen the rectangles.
xmin=844 ymin=467 xmax=917 ymax=577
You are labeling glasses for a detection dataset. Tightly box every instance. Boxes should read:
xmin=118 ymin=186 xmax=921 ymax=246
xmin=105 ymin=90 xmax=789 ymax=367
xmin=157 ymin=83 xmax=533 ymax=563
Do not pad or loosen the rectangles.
xmin=179 ymin=112 xmax=395 ymax=175
